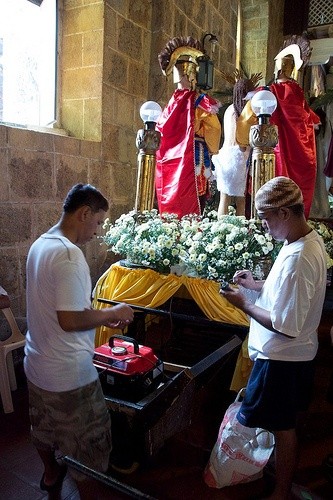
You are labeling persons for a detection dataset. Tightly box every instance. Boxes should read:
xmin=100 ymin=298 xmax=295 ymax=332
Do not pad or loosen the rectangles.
xmin=218 ymin=176 xmax=327 ymax=500
xmin=154 ymin=34 xmax=321 ymax=240
xmin=24 ymin=183 xmax=135 ymax=500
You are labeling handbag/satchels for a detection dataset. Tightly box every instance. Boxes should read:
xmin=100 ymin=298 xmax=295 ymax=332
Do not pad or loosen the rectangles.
xmin=203 ymin=387 xmax=275 ymax=489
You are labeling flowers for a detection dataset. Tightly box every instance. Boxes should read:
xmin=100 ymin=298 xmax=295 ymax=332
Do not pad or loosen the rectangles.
xmin=97 ymin=205 xmax=333 ymax=282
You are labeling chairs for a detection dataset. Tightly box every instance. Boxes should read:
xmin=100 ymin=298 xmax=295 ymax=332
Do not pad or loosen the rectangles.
xmin=0 ymin=305 xmax=26 ymax=414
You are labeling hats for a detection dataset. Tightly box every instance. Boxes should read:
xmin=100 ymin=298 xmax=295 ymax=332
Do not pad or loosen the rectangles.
xmin=255 ymin=176 xmax=303 ymax=213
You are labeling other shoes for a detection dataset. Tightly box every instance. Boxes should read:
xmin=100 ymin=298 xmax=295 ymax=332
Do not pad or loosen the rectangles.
xmin=40 ymin=455 xmax=68 ymax=490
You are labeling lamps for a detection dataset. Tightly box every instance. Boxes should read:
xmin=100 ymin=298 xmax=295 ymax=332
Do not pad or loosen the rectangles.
xmin=134 ymin=100 xmax=162 ymax=212
xmin=249 ymin=90 xmax=278 ymax=219
